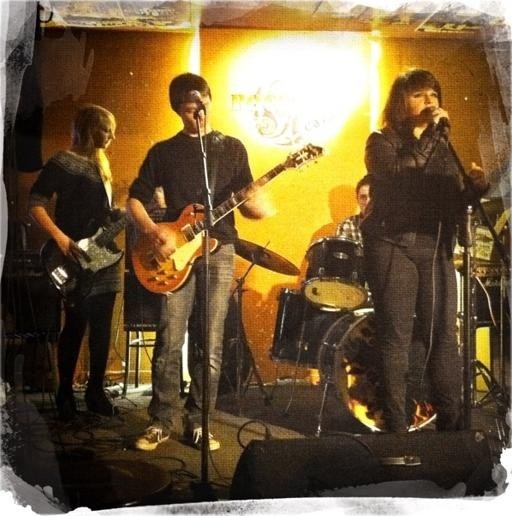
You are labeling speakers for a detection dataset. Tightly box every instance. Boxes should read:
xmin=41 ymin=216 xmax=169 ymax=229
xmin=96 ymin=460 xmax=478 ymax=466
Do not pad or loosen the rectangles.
xmin=228 ymin=431 xmax=496 ymax=496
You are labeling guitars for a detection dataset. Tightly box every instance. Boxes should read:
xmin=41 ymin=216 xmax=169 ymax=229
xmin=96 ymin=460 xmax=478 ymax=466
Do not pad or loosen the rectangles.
xmin=39 ymin=218 xmax=127 ymax=300
xmin=131 ymin=144 xmax=325 ymax=293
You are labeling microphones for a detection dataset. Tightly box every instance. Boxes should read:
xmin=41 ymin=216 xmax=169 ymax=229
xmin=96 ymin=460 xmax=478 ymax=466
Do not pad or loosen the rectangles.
xmin=190 ymin=89 xmax=206 ymax=115
xmin=426 ymin=106 xmax=451 ymax=128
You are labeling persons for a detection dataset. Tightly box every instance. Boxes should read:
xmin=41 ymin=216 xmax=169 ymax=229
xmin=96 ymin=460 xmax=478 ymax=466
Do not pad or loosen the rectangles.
xmin=27 ymin=102 xmax=161 ymax=420
xmin=124 ymin=71 xmax=268 ymax=453
xmin=336 ymin=172 xmax=373 ymax=246
xmin=358 ymin=68 xmax=490 ymax=434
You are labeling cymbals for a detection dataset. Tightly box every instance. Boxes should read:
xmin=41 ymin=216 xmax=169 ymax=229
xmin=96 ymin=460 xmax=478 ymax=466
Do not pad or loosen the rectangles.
xmin=234 ymin=239 xmax=300 ymax=276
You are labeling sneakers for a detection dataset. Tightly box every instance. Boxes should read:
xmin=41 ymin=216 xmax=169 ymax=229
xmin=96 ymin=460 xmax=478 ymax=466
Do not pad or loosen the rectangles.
xmin=85 ymin=388 xmax=119 ymax=416
xmin=136 ymin=426 xmax=169 ymax=451
xmin=55 ymin=385 xmax=76 ymax=414
xmin=191 ymin=427 xmax=220 ymax=451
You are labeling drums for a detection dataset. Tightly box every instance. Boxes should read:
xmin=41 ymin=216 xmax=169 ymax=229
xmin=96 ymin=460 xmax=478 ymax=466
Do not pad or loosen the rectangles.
xmin=302 ymin=237 xmax=369 ymax=311
xmin=269 ymin=288 xmax=347 ymax=365
xmin=316 ymin=308 xmax=440 ymax=434
